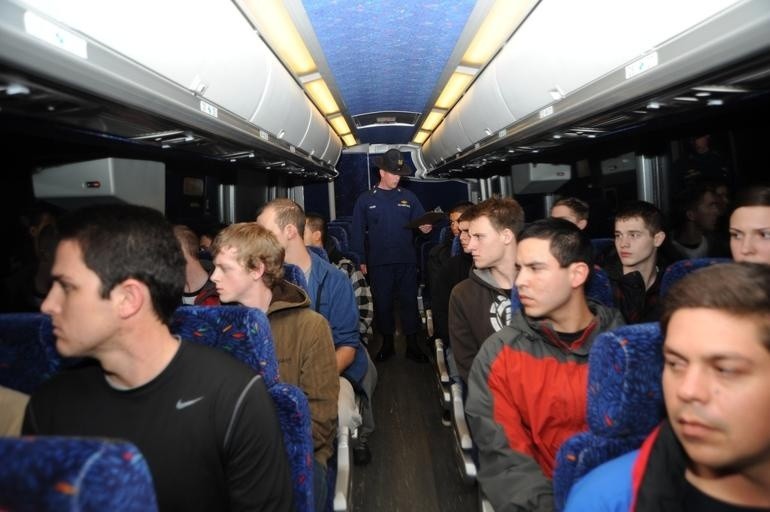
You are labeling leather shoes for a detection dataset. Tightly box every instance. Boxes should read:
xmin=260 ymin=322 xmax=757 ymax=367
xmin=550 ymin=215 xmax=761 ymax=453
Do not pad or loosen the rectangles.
xmin=377 ymin=343 xmax=395 ymax=361
xmin=405 ymin=345 xmax=428 ymax=364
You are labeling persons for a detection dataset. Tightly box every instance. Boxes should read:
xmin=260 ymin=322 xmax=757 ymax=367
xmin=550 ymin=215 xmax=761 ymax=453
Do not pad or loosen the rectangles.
xmin=20 ymin=205 xmax=290 ymax=510
xmin=444 ymin=205 xmax=478 ymax=295
xmin=303 ymin=216 xmax=378 ymax=466
xmin=428 ymin=201 xmax=475 ymax=282
xmin=447 ymin=196 xmax=524 ymax=384
xmin=352 ymin=148 xmax=432 ymax=364
xmin=199 ymin=232 xmax=212 ymax=252
xmin=28 ymin=211 xmax=59 ymax=308
xmin=719 ymin=194 xmax=770 ymax=265
xmin=210 ymin=222 xmax=340 ymax=510
xmin=549 ymin=197 xmax=589 ymax=230
xmin=172 ymin=225 xmax=221 ymax=306
xmin=564 ymin=262 xmax=770 ymax=512
xmin=609 ymin=200 xmax=665 ymax=324
xmin=663 ymin=185 xmax=719 ymax=263
xmin=254 ymin=198 xmax=368 ymax=511
xmin=465 ymin=217 xmax=626 ymax=512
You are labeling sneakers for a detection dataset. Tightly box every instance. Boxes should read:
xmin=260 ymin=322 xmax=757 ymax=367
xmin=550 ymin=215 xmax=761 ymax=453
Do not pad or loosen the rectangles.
xmin=442 ymin=410 xmax=451 ymax=426
xmin=355 ymin=443 xmax=371 ymax=469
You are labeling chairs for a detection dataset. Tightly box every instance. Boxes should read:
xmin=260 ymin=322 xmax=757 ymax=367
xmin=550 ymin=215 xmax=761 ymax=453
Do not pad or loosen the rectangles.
xmin=0 ymin=439 xmax=155 ymax=512
xmin=306 ymin=246 xmax=329 ymax=265
xmin=168 ymin=306 xmax=314 ymax=510
xmin=591 ymin=233 xmax=638 ymax=273
xmin=659 ymin=254 xmax=732 ymax=300
xmin=481 ymin=270 xmax=617 ymax=511
xmin=282 ymin=264 xmax=361 ymax=511
xmin=446 ymin=384 xmax=474 ymax=489
xmin=331 ymin=223 xmax=357 ymax=249
xmin=0 ymin=313 xmax=61 ymax=385
xmin=551 ymin=320 xmax=664 ymax=505
xmin=331 ymin=227 xmax=347 ymax=252
xmin=433 ymin=236 xmax=464 ymax=412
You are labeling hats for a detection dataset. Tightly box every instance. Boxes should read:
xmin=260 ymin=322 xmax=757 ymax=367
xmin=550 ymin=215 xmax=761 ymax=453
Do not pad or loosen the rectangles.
xmin=372 ymin=149 xmax=411 ymax=175
